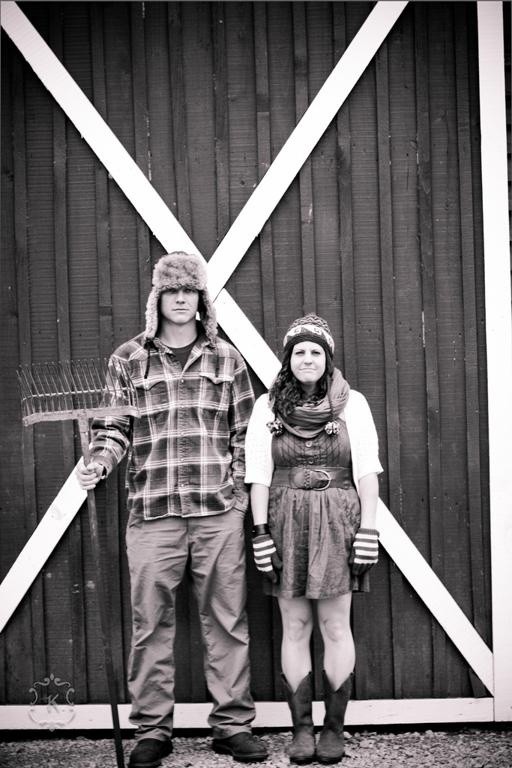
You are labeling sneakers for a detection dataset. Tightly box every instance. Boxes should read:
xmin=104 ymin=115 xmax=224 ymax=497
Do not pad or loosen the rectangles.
xmin=129 ymin=739 xmax=173 ymax=768
xmin=213 ymin=732 xmax=269 ymax=760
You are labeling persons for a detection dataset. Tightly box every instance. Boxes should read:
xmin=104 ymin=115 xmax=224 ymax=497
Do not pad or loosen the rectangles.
xmin=241 ymin=312 xmax=386 ymax=767
xmin=73 ymin=249 xmax=269 ymax=766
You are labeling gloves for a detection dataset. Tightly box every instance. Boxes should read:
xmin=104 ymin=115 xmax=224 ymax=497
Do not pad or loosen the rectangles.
xmin=348 ymin=529 xmax=379 ymax=577
xmin=252 ymin=534 xmax=282 ymax=583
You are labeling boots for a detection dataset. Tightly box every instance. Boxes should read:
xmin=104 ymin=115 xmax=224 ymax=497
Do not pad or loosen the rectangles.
xmin=277 ymin=670 xmax=315 ymax=762
xmin=316 ymin=670 xmax=356 ymax=762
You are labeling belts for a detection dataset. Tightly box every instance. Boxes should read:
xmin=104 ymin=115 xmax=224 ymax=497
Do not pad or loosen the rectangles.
xmin=272 ymin=466 xmax=351 ymax=489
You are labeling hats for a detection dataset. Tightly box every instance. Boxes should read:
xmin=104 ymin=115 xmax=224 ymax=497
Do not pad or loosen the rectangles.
xmin=143 ymin=253 xmax=216 ymax=347
xmin=283 ymin=313 xmax=335 ymax=353
